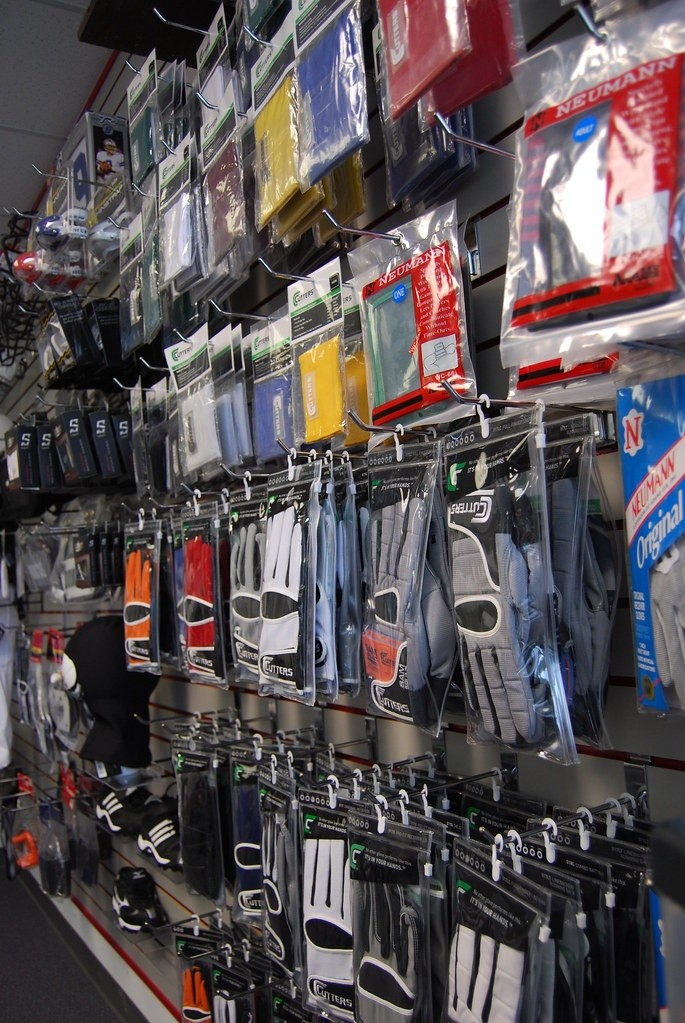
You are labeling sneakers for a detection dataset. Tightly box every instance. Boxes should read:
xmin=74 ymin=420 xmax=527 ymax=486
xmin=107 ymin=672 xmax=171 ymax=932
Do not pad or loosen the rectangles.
xmin=112 ymin=866 xmax=170 ymax=935
xmin=136 ymin=794 xmax=180 ymax=869
xmin=95 ymin=779 xmax=155 ymax=836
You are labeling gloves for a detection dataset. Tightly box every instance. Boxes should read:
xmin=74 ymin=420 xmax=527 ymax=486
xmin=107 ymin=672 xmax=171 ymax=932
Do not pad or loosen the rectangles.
xmin=123 ymin=399 xmax=659 ymax=1023
